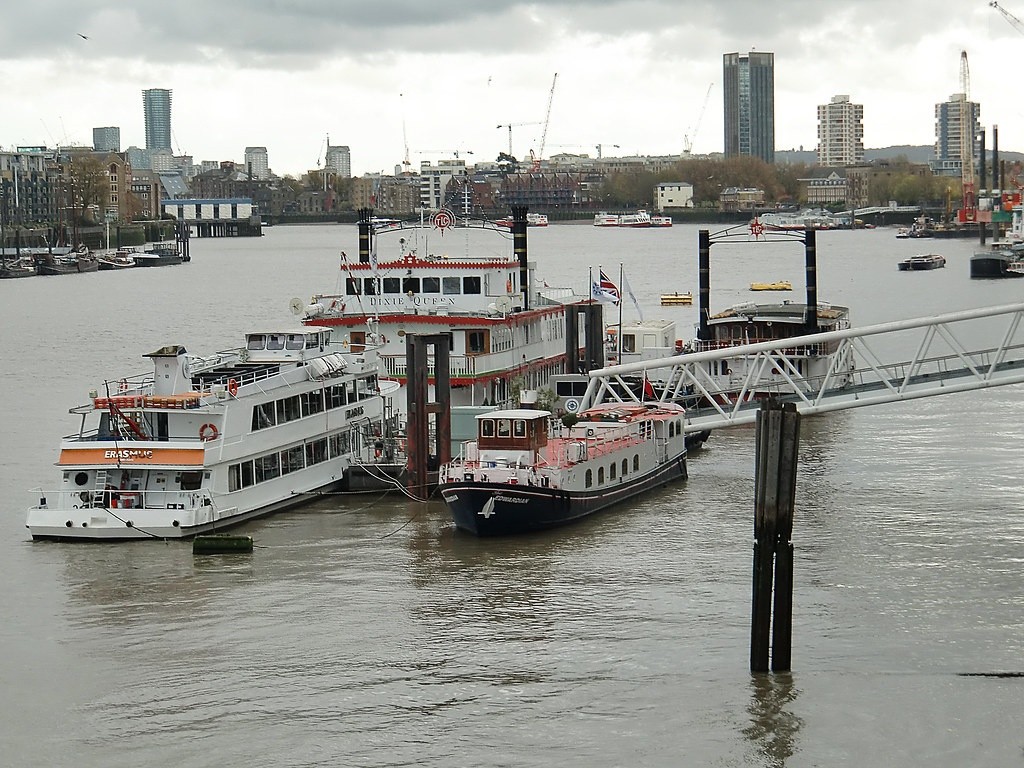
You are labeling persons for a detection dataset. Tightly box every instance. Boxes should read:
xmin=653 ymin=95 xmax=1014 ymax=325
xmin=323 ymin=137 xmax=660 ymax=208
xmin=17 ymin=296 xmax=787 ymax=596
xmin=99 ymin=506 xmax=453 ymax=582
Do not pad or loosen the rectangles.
xmin=373 ymin=428 xmax=382 ymax=457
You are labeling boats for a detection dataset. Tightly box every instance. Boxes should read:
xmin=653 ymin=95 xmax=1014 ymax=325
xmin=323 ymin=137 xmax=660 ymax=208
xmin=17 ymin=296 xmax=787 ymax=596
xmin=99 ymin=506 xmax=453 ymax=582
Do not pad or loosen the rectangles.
xmin=21 ymin=326 xmax=400 ymax=544
xmin=95 ymin=252 xmax=136 ymax=269
xmin=32 ymin=252 xmax=99 ymax=275
xmin=749 ymin=279 xmax=793 ymax=292
xmin=354 ymin=215 xmax=403 ymax=228
xmin=649 ymin=215 xmax=673 ymax=227
xmin=0 ymin=252 xmax=39 ymax=278
xmin=896 ymin=215 xmax=940 ymax=239
xmin=601 ymin=308 xmax=688 ymax=396
xmin=494 ymin=215 xmax=515 ymax=227
xmin=746 ymin=206 xmax=876 ymax=230
xmin=674 ymin=214 xmax=857 ymax=406
xmin=970 ymin=211 xmax=1024 ymax=279
xmin=592 ymin=212 xmax=620 ymax=227
xmin=132 ymin=243 xmax=184 ymax=267
xmin=289 ymin=198 xmax=608 ymax=470
xmin=897 ymin=254 xmax=947 ymax=271
xmin=436 ymin=396 xmax=693 ymax=540
xmin=525 ymin=213 xmax=549 ymax=227
xmin=617 ymin=209 xmax=651 ymax=228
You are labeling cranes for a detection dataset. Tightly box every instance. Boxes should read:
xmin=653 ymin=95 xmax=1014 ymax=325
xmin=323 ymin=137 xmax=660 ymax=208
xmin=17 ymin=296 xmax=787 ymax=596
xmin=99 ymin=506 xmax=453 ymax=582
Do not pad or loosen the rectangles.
xmin=535 ymin=71 xmax=560 ymax=161
xmin=677 ymin=81 xmax=718 ymax=157
xmin=412 ymin=147 xmax=476 ymax=161
xmin=545 ymin=142 xmax=620 ymax=161
xmin=400 ymin=119 xmax=411 ymax=177
xmin=496 ymin=120 xmax=550 ymax=153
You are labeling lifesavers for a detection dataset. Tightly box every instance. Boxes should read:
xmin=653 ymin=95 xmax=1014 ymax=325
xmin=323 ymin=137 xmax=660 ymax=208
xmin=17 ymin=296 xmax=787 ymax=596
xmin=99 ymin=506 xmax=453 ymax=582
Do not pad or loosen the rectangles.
xmin=199 ymin=424 xmax=218 ymax=441
xmin=398 ymin=431 xmax=404 ymax=450
xmin=507 ymin=280 xmax=511 ymax=292
xmin=229 ymin=378 xmax=237 ymax=396
xmin=817 ymin=309 xmax=838 ymax=318
xmin=332 ymin=299 xmax=344 ymax=311
xmin=1014 ymin=234 xmax=1018 ymax=238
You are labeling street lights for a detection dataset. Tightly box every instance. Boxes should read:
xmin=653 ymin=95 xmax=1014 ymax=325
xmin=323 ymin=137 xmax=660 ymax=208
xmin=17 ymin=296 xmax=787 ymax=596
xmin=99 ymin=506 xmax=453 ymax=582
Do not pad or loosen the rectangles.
xmin=10 ymin=162 xmax=21 ymax=222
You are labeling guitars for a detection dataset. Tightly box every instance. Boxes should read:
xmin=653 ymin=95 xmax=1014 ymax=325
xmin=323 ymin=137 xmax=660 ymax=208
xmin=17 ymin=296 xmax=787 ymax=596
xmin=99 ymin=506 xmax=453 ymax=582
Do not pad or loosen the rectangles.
xmin=120 ymin=383 xmax=128 ymax=392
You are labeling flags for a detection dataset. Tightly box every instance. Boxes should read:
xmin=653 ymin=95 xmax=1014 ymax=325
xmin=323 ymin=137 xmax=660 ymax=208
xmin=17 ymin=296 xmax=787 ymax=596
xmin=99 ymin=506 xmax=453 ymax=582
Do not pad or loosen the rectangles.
xmin=600 ymin=269 xmax=620 ymax=307
xmin=591 ymin=274 xmax=619 ymax=303
xmin=623 ymin=269 xmax=643 ymax=322
xmin=645 ymin=372 xmax=652 ymax=399
